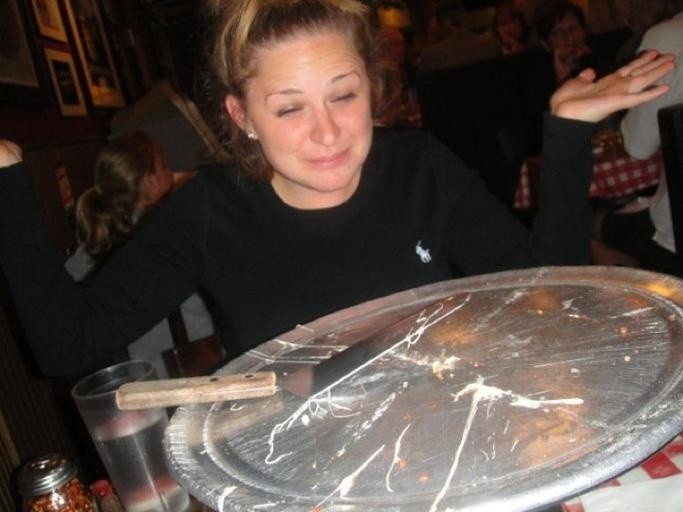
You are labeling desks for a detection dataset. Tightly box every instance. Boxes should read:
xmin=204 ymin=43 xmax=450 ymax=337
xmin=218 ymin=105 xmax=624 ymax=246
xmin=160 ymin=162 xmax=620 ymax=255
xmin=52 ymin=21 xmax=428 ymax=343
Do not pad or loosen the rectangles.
xmin=523 ymin=128 xmax=663 ymax=206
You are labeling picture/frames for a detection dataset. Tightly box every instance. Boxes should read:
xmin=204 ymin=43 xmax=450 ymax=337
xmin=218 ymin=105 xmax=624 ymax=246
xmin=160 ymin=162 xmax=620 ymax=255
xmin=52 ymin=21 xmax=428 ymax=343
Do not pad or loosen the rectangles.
xmin=2 ymin=1 xmax=134 ymax=121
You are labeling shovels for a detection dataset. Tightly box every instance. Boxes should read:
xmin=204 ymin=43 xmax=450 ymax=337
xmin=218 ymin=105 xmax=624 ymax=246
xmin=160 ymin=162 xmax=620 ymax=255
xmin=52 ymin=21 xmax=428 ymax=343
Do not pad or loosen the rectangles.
xmin=116 ymin=292 xmax=473 ymax=412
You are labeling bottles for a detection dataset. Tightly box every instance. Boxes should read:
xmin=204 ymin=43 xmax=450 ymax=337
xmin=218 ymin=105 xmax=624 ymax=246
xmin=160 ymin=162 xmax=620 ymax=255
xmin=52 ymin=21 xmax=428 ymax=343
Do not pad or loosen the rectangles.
xmin=90 ymin=480 xmax=126 ymax=512
xmin=15 ymin=452 xmax=99 ymax=512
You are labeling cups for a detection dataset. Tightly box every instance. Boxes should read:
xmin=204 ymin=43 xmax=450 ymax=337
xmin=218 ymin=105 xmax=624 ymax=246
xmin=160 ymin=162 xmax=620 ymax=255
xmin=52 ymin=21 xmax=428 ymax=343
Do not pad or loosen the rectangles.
xmin=70 ymin=359 xmax=191 ymax=512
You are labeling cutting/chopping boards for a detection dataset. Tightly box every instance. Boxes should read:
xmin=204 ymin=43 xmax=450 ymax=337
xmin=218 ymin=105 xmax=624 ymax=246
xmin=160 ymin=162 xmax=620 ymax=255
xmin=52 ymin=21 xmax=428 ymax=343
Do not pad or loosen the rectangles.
xmin=161 ymin=265 xmax=682 ymax=512
